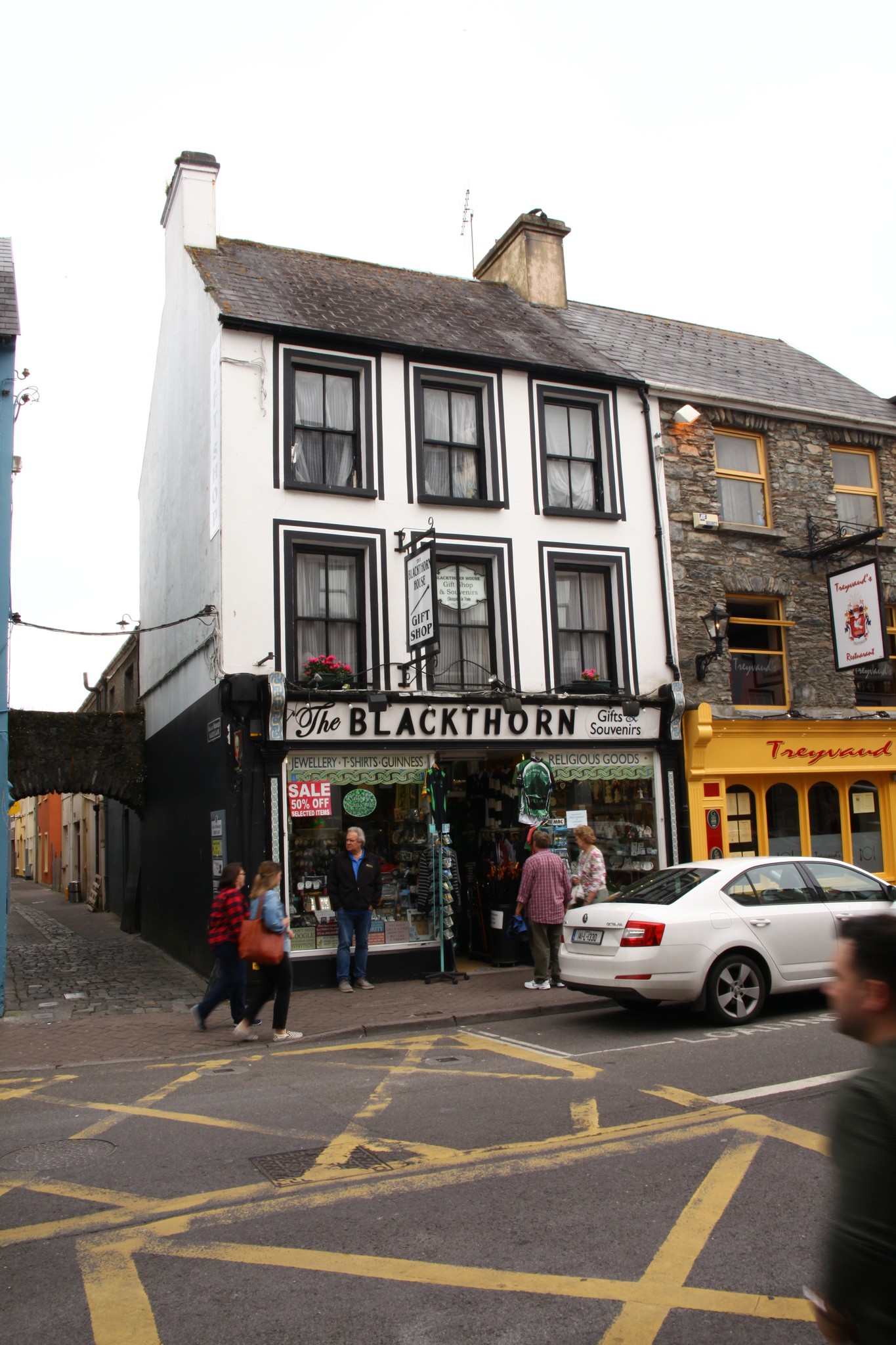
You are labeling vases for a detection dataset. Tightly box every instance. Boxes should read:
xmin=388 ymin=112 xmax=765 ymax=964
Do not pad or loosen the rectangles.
xmin=571 ymin=680 xmax=611 ymax=693
xmin=312 ymin=672 xmax=341 ymax=688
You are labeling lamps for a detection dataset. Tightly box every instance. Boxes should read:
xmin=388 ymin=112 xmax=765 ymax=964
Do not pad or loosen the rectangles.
xmin=502 ymin=692 xmax=523 ymax=714
xmin=307 ymin=673 xmax=322 ymax=689
xmin=673 ymin=404 xmax=702 ymax=424
xmin=116 ymin=614 xmax=140 ymax=630
xmin=367 ymin=695 xmax=387 ymax=712
xmin=488 ymin=674 xmax=506 ymax=691
xmin=787 ymin=710 xmax=798 ymax=718
xmin=622 ymin=694 xmax=640 ymax=717
xmin=696 ymin=600 xmax=730 ymax=681
xmin=874 ymin=711 xmax=890 ymax=718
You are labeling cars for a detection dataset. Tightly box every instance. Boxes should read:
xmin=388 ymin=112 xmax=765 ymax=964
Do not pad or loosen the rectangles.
xmin=557 ymin=855 xmax=896 ymax=1025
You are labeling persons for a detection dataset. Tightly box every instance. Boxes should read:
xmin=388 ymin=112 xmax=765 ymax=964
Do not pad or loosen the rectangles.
xmin=327 ymin=826 xmax=381 ymax=993
xmin=571 ymin=826 xmax=609 ymax=907
xmin=192 ymin=862 xmax=261 ymax=1031
xmin=515 ymin=830 xmax=573 ymax=991
xmin=231 ymin=861 xmax=303 ymax=1041
xmin=808 ymin=916 xmax=895 ymax=1345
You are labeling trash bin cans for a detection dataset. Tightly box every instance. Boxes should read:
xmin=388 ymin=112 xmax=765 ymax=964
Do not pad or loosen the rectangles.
xmin=68 ymin=881 xmax=81 ymax=902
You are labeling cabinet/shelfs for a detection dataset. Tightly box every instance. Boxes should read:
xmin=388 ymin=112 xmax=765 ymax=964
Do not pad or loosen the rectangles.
xmin=572 ymin=780 xmax=657 ymax=882
xmin=389 ymin=793 xmax=470 ymax=985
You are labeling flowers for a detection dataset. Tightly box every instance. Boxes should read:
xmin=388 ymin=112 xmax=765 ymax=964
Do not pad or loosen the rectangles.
xmin=301 ymin=653 xmax=353 ymax=674
xmin=579 ymin=669 xmax=599 ymax=680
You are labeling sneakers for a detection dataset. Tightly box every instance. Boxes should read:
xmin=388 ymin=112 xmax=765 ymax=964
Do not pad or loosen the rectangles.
xmin=273 ymin=1030 xmax=303 ymax=1042
xmin=233 ymin=1027 xmax=258 ymax=1041
xmin=525 ymin=979 xmax=551 ymax=990
xmin=354 ymin=977 xmax=374 ymax=989
xmin=338 ymin=979 xmax=354 ymax=993
xmin=549 ymin=978 xmax=565 ymax=988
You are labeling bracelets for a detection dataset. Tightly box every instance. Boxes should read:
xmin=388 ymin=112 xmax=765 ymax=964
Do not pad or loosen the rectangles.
xmin=584 ymin=900 xmax=591 ymax=905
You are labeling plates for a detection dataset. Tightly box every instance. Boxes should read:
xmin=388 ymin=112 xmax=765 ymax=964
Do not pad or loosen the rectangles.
xmin=609 ymin=855 xmax=623 ymax=866
xmin=641 ymin=862 xmax=654 ymax=871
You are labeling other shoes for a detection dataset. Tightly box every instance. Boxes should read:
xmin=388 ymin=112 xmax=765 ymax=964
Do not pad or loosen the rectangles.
xmin=233 ymin=1019 xmax=262 ymax=1027
xmin=190 ymin=1004 xmax=207 ymax=1033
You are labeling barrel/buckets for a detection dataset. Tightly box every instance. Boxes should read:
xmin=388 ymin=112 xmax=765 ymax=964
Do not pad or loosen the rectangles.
xmin=490 ymin=902 xmax=521 ymax=967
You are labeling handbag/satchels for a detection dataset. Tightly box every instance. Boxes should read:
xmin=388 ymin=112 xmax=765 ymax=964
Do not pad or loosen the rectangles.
xmin=237 ymin=889 xmax=285 ymax=964
